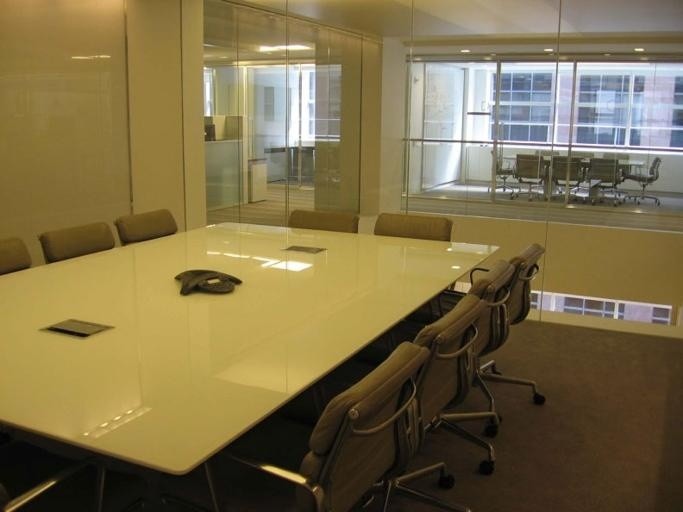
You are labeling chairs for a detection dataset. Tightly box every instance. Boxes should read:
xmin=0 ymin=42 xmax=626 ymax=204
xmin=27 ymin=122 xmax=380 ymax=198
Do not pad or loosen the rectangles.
xmin=488 ymin=148 xmax=662 ymax=209
